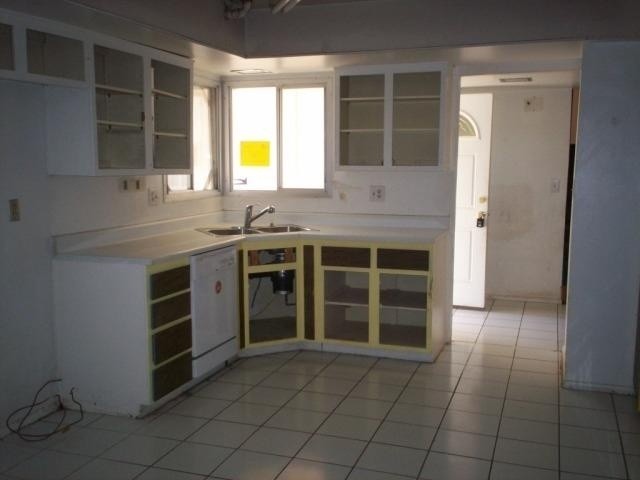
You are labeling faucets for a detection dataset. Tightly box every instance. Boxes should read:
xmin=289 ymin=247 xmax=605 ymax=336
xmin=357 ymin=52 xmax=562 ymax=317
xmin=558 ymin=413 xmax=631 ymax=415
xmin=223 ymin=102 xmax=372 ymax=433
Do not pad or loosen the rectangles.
xmin=243 ymin=203 xmax=275 ymax=228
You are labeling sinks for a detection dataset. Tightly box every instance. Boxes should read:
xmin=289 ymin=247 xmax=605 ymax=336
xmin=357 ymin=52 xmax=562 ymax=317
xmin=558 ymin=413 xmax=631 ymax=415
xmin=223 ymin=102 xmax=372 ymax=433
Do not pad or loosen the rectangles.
xmin=208 ymin=229 xmax=260 ymax=235
xmin=255 ymin=226 xmax=312 ymax=233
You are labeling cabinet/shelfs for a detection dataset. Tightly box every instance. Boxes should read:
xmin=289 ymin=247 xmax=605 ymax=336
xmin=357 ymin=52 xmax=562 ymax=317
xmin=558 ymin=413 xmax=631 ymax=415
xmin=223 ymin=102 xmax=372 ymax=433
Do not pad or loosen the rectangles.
xmin=57 ymin=226 xmax=448 ymax=418
